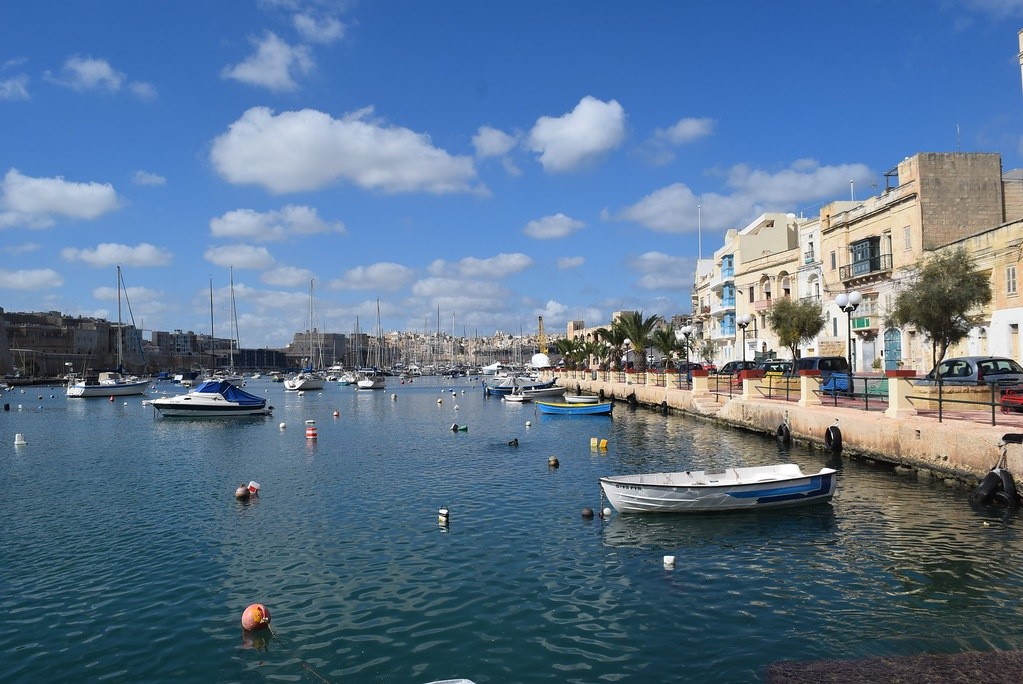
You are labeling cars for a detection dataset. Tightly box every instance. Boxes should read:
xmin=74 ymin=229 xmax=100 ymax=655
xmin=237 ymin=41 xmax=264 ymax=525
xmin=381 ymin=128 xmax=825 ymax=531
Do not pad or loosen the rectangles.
xmin=783 ymin=356 xmax=849 ymax=396
xmin=646 ymin=359 xmax=703 ymax=381
xmin=914 ymin=356 xmax=1023 ymax=395
xmin=713 ymin=357 xmax=793 ymax=376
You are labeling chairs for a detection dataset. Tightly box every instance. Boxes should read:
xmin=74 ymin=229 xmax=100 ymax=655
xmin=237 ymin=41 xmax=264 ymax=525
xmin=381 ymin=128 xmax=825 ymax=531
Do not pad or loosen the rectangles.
xmin=960 ymin=368 xmax=970 ymax=376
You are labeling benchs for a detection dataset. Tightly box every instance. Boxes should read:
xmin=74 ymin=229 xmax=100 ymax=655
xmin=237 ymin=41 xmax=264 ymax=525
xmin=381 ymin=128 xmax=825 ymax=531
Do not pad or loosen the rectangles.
xmin=727 ymin=375 xmax=743 ymax=390
xmin=820 ymin=370 xmax=855 ymax=398
xmin=861 ymin=373 xmax=888 ymax=402
xmin=1000 ymin=385 xmax=1023 ymax=415
xmin=764 ymin=371 xmax=783 ymax=379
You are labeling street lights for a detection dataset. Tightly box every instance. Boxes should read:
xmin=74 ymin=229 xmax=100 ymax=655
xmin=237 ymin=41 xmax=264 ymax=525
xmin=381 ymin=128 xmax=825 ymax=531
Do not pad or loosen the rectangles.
xmin=835 ymin=290 xmax=862 ymax=400
xmin=681 ymin=325 xmax=692 ymax=375
xmin=735 ymin=313 xmax=752 ymax=370
xmin=605 ymin=342 xmax=611 ymax=372
xmin=623 ymin=339 xmax=631 ymax=369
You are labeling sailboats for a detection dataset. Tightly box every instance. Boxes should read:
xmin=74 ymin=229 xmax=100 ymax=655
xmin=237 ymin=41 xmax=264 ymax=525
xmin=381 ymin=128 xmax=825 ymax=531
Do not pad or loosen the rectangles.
xmin=65 ymin=265 xmax=153 ymax=398
xmin=317 ymin=296 xmax=564 ymax=390
xmin=158 ymin=264 xmax=245 ymax=387
xmin=283 ymin=278 xmax=326 ymax=390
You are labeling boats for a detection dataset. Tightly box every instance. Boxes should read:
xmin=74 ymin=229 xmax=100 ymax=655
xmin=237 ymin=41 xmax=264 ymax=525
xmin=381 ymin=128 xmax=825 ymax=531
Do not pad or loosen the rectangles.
xmin=3 ymin=365 xmax=30 ymax=387
xmin=599 ymin=464 xmax=841 ymax=516
xmin=241 ymin=371 xmax=297 ymax=381
xmin=536 ymin=399 xmax=613 ymax=414
xmin=481 ymin=374 xmax=558 ymax=396
xmin=511 ymin=384 xmax=568 ymax=397
xmin=503 ymin=395 xmax=534 ymax=401
xmin=564 ymin=394 xmax=599 ymax=403
xmin=142 ymin=377 xmax=273 ymax=417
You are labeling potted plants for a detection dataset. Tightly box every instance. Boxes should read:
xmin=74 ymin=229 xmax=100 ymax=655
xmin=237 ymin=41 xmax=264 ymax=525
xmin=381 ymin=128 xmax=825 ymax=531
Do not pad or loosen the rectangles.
xmin=871 ymin=357 xmax=882 ymax=373
